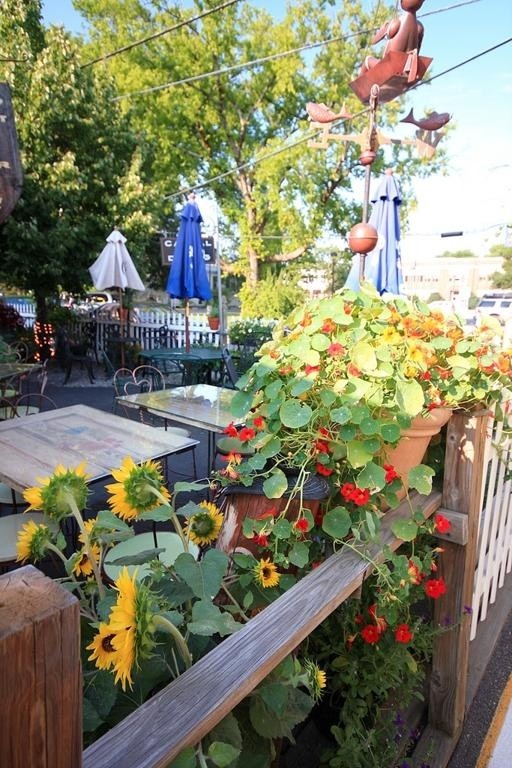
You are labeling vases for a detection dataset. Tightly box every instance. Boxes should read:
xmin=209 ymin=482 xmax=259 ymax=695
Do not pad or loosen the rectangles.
xmin=359 ymin=406 xmax=453 ymax=514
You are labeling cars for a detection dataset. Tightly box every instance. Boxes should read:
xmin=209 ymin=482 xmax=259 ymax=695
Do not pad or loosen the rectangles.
xmin=82 ymin=301 xmax=215 ymax=351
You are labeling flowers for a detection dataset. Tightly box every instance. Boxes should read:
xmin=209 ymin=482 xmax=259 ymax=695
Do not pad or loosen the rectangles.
xmin=209 ymin=278 xmax=512 ymax=651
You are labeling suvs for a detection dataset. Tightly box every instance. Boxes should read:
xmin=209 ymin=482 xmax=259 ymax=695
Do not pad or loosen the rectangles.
xmin=475 ymin=292 xmax=511 ymax=329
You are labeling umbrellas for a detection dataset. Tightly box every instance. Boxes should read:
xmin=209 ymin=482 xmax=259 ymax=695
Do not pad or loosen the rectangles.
xmin=166 ymin=193 xmax=212 ymax=351
xmin=342 ymin=169 xmax=405 ymax=295
xmin=86 ymin=224 xmax=147 ymax=376
xmin=0 ymin=82 xmax=25 ymax=221
